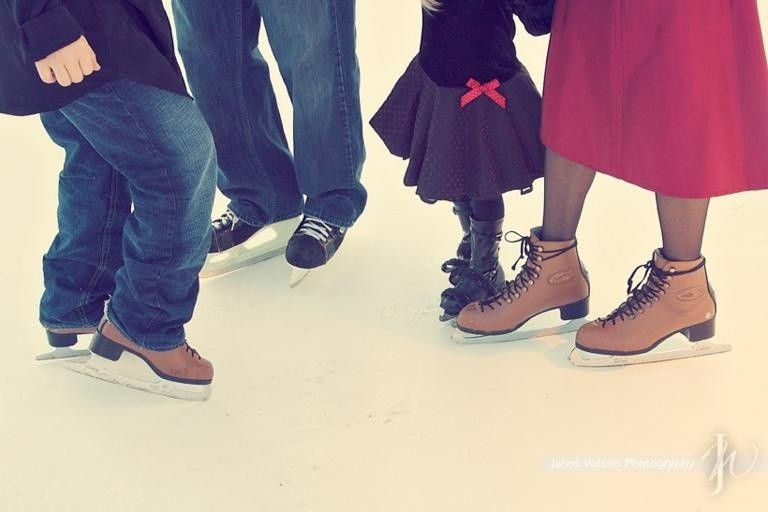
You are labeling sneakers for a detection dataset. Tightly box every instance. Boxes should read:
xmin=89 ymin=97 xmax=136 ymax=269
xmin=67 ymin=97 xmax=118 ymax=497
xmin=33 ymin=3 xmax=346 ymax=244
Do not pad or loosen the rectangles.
xmin=207 ymin=208 xmax=254 ymax=255
xmin=284 ymin=214 xmax=348 ymax=271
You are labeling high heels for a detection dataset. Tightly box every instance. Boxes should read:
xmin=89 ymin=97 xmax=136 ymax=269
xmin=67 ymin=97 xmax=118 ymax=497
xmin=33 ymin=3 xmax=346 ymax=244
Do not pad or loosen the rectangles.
xmin=45 ymin=315 xmax=214 ymax=385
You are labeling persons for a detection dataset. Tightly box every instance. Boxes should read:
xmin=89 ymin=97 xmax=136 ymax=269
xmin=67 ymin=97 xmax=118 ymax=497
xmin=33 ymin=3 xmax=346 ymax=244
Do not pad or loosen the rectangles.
xmin=368 ymin=1 xmax=554 ymax=322
xmin=0 ymin=1 xmax=218 ymax=403
xmin=450 ymin=0 xmax=767 ymax=367
xmin=170 ymin=1 xmax=368 ymax=288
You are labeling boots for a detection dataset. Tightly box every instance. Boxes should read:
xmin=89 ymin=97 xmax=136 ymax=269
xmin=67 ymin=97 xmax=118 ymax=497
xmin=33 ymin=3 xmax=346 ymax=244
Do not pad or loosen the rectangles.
xmin=454 ymin=226 xmax=589 ymax=336
xmin=436 ymin=201 xmax=507 ymax=316
xmin=574 ymin=246 xmax=716 ymax=357
